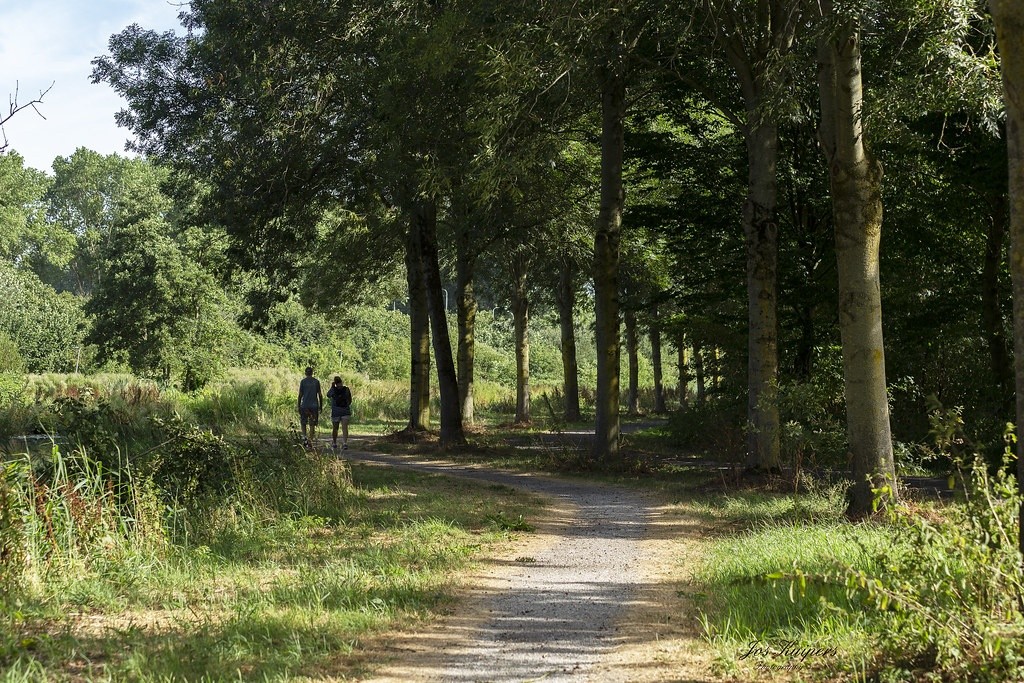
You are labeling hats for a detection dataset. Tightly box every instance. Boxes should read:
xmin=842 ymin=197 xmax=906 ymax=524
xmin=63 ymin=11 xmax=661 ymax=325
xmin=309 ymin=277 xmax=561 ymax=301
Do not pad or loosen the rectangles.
xmin=305 ymin=366 xmax=313 ymax=372
xmin=334 ymin=376 xmax=341 ymax=381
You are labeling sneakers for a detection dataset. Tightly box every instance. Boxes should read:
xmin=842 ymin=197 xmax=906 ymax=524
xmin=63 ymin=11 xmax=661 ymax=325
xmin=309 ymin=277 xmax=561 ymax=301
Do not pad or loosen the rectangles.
xmin=331 ymin=444 xmax=337 ymax=448
xmin=340 ymin=444 xmax=348 ymax=450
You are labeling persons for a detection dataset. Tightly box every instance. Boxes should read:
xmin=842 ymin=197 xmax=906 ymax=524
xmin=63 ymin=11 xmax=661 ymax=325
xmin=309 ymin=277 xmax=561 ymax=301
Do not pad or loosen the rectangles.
xmin=327 ymin=377 xmax=352 ymax=450
xmin=297 ymin=368 xmax=323 ymax=437
